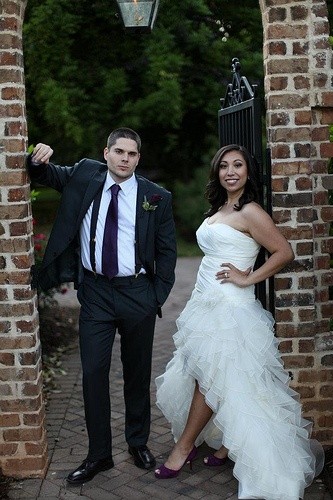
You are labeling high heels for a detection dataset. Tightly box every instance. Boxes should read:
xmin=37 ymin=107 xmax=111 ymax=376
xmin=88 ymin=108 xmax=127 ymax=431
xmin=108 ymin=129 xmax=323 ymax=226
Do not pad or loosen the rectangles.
xmin=203 ymin=453 xmax=229 ymax=467
xmin=154 ymin=444 xmax=197 ymax=478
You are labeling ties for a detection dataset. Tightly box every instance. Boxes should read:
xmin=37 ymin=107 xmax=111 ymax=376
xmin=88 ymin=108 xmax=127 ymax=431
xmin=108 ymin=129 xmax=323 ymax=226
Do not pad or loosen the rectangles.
xmin=101 ymin=183 xmax=122 ymax=279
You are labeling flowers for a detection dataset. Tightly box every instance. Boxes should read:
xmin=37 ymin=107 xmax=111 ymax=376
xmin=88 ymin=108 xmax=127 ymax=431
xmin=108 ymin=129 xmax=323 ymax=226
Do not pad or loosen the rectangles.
xmin=142 ymin=194 xmax=163 ymax=211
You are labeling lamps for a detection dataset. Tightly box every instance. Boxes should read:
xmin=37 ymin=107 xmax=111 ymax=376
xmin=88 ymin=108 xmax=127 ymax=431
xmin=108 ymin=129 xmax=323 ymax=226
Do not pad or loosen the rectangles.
xmin=114 ymin=0 xmax=160 ymax=34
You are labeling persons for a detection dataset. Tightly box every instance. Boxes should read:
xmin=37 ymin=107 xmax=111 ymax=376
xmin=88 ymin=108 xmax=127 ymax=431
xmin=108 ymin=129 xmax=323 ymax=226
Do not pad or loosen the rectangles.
xmin=27 ymin=128 xmax=177 ymax=485
xmin=153 ymin=143 xmax=295 ymax=478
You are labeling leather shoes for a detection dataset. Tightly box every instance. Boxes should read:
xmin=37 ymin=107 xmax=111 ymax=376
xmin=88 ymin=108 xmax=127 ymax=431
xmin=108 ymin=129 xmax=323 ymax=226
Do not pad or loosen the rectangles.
xmin=127 ymin=449 xmax=156 ymax=469
xmin=65 ymin=455 xmax=114 ymax=483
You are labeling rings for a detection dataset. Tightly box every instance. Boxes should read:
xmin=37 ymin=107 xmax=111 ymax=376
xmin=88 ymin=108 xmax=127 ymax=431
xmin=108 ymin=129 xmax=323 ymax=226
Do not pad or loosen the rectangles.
xmin=224 ymin=272 xmax=228 ymax=278
